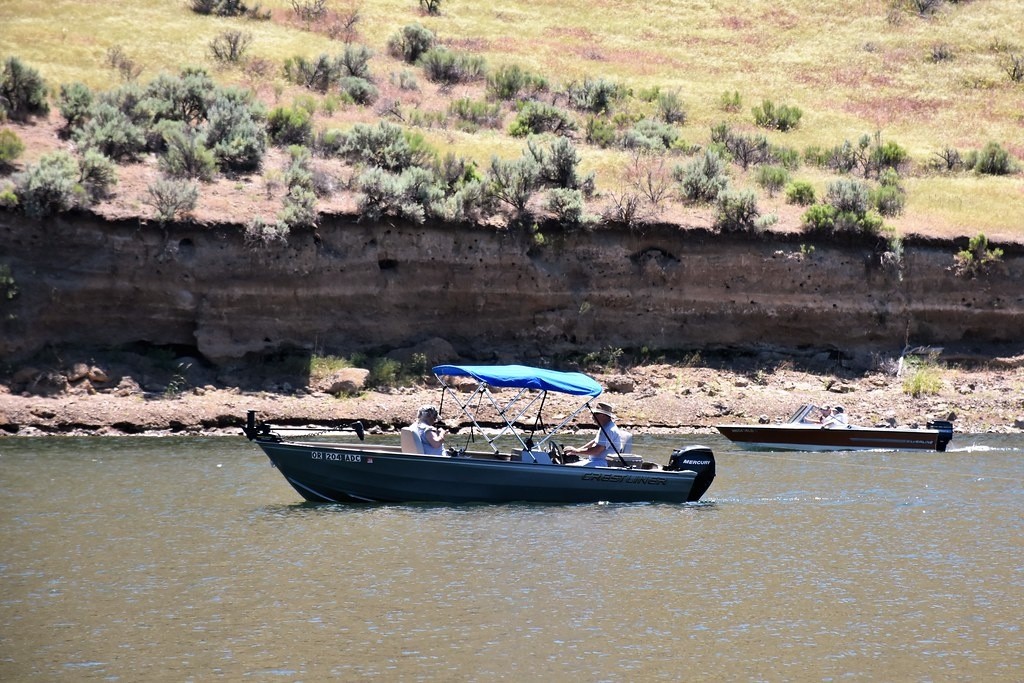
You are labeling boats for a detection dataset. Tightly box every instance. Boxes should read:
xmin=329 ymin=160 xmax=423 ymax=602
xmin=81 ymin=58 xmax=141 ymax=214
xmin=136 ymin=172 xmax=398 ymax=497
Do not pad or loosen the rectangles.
xmin=231 ymin=364 xmax=716 ymax=501
xmin=713 ymin=403 xmax=952 ymax=454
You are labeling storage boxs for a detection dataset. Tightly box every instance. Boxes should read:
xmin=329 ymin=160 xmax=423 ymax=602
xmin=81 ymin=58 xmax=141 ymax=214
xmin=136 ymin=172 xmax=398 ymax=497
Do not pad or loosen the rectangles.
xmin=605 ymin=454 xmax=643 ymax=469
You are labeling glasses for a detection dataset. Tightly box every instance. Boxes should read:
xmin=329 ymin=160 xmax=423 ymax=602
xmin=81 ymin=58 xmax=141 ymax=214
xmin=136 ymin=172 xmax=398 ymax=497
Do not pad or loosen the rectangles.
xmin=593 ymin=412 xmax=603 ymax=416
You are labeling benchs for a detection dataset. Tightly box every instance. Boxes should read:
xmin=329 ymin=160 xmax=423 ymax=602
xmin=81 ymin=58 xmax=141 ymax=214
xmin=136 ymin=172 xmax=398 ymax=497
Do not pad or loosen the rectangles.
xmin=401 ymin=427 xmax=424 ymax=454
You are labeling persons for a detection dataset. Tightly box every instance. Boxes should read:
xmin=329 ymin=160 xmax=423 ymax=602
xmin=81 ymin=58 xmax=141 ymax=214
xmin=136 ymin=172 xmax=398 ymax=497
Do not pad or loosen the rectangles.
xmin=807 ymin=406 xmax=845 ymax=429
xmin=563 ymin=402 xmax=622 ymax=467
xmin=410 ymin=406 xmax=449 ymax=456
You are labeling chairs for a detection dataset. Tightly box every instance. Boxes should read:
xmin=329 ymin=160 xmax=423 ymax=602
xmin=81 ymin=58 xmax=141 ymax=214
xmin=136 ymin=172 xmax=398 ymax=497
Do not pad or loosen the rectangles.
xmin=619 ymin=431 xmax=633 ymax=452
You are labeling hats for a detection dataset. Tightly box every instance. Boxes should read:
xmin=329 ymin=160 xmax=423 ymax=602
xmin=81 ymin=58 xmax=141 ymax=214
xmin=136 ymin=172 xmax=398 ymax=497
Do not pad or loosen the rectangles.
xmin=589 ymin=402 xmax=615 ymax=419
xmin=418 ymin=405 xmax=442 ymax=420
